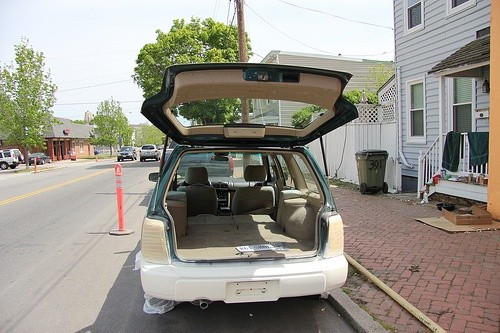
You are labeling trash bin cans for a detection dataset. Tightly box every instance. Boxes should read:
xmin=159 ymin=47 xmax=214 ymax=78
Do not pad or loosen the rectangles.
xmin=355 ymin=149 xmax=388 ymax=191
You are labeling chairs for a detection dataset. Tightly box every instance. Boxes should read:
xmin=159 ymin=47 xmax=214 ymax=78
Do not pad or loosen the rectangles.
xmin=232 ymin=164 xmax=274 ymax=214
xmin=177 ymin=166 xmax=218 ymax=216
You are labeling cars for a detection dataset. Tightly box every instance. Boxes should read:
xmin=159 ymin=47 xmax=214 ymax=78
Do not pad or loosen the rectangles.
xmin=29 ymin=152 xmax=52 ymax=165
xmin=94 ymin=150 xmax=99 ymax=155
xmin=138 ymin=61 xmax=360 ymax=310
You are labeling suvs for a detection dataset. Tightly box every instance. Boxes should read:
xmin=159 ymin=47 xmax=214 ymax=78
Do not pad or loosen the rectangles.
xmin=116 ymin=146 xmax=138 ymax=162
xmin=160 ymin=136 xmax=234 ymax=179
xmin=0 ymin=148 xmax=24 ymax=170
xmin=139 ymin=145 xmax=162 ymax=162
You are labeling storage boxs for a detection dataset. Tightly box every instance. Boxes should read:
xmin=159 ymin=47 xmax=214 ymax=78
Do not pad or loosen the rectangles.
xmin=442 ymin=206 xmax=493 ymax=225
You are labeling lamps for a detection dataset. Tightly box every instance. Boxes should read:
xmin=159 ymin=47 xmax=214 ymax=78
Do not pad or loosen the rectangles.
xmin=482 ymin=80 xmax=489 ymax=93
